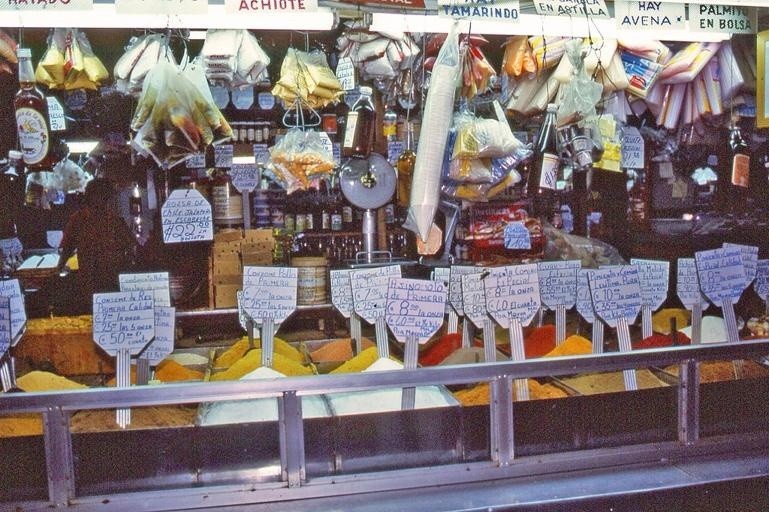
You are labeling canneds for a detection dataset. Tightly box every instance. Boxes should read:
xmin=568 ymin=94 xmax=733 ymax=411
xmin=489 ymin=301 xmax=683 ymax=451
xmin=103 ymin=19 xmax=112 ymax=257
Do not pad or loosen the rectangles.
xmin=229 ymin=122 xmax=270 ymax=144
xmin=252 ymin=188 xmax=287 ymax=228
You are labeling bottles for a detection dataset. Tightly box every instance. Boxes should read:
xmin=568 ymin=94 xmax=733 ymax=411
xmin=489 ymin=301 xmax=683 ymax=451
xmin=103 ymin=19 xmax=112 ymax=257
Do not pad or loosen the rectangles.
xmin=13 ymin=49 xmax=52 ymax=171
xmin=721 ymin=126 xmax=749 ymax=205
xmin=398 ymin=122 xmax=416 ymax=205
xmin=284 ymin=189 xmax=364 ymax=262
xmin=527 ymin=105 xmax=561 ymax=203
xmin=227 ymin=122 xmax=271 ymax=144
xmin=341 ymin=84 xmax=377 ymax=158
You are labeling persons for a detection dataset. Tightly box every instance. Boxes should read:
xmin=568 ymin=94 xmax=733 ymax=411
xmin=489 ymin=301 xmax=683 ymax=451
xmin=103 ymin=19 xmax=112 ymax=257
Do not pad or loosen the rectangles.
xmin=56 ymin=177 xmax=139 ymax=313
